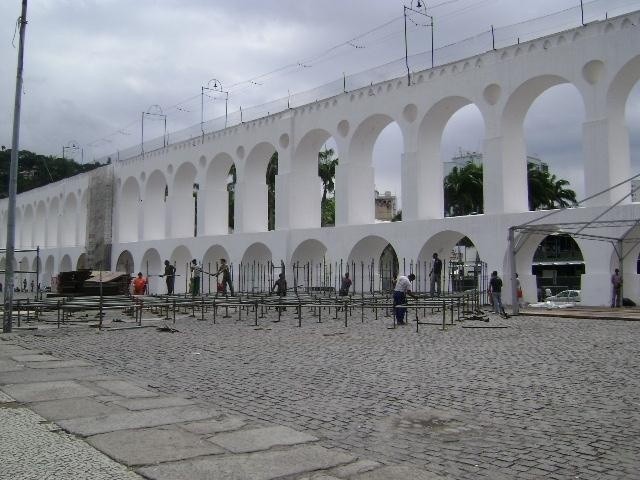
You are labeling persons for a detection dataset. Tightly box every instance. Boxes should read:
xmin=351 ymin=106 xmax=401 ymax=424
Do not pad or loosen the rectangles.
xmin=487 ymin=270 xmax=505 ymax=314
xmin=160 ymin=260 xmax=176 ymax=296
xmin=429 ymin=252 xmax=442 ymax=297
xmin=340 ymin=271 xmax=353 ymax=296
xmin=133 ymin=272 xmax=148 ymax=296
xmin=391 ymin=276 xmax=397 ymax=288
xmin=272 ymin=272 xmax=288 ymax=310
xmin=189 ymin=276 xmax=195 ymax=293
xmin=515 ymin=273 xmax=524 ymax=309
xmin=190 ymin=259 xmax=201 ymax=296
xmin=212 ymin=258 xmax=234 ymax=296
xmin=394 ymin=274 xmax=418 ymax=324
xmin=611 ymin=269 xmax=624 ymax=311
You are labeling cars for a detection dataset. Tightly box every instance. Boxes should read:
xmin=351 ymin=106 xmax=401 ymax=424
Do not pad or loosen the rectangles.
xmin=544 ymin=289 xmax=580 ymax=302
